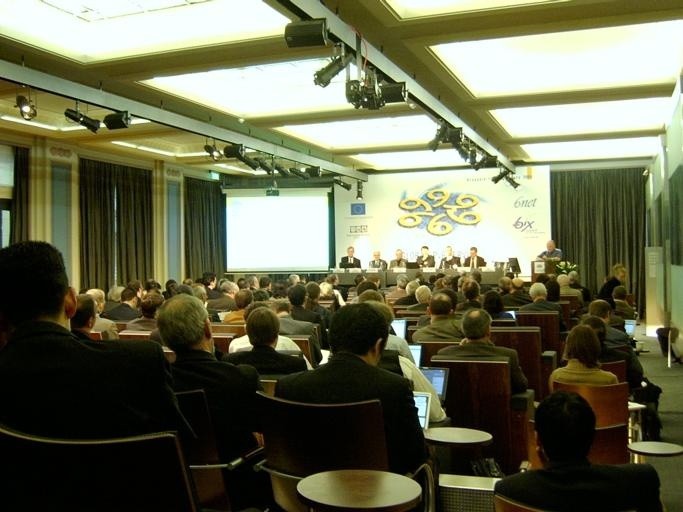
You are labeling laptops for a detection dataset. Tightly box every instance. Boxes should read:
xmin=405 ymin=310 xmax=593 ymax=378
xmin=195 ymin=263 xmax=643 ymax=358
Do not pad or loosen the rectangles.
xmin=390 ymin=319 xmax=407 ymax=339
xmin=505 ymin=311 xmax=516 ymax=320
xmin=419 ymin=366 xmax=450 ymax=412
xmin=624 ymin=320 xmax=636 ymax=339
xmin=413 ymin=391 xmax=432 ymax=431
xmin=408 ymin=344 xmax=422 ymax=368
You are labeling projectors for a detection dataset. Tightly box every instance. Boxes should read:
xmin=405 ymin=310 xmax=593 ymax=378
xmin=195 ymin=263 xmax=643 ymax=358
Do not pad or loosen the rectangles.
xmin=265 ymin=185 xmax=279 ymax=197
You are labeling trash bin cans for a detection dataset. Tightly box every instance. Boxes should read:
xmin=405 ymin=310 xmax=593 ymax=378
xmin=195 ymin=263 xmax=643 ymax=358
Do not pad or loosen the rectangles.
xmin=655 ymin=328 xmax=680 ymax=357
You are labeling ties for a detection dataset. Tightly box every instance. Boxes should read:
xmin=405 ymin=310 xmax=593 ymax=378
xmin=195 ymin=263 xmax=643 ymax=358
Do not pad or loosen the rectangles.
xmin=471 ymin=258 xmax=473 ymax=268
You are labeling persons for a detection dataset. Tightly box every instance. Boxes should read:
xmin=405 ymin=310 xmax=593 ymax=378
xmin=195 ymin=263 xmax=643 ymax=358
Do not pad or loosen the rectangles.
xmin=439 ymin=246 xmax=461 ymax=269
xmin=577 ymin=299 xmax=661 ymax=441
xmin=464 ymin=246 xmax=486 ymax=267
xmin=155 ymin=293 xmax=266 ymax=511
xmin=536 ymin=239 xmax=564 ymax=262
xmin=493 ymin=385 xmax=665 ymax=512
xmin=368 ymin=249 xmax=388 ymax=270
xmin=271 ymin=299 xmax=431 ymax=481
xmin=416 ymin=245 xmax=437 ymax=266
xmin=70 ymin=261 xmax=665 ymax=386
xmin=340 ymin=246 xmax=360 ymax=269
xmin=0 ymin=239 xmax=199 ymax=510
xmin=391 ymin=248 xmax=408 ymax=268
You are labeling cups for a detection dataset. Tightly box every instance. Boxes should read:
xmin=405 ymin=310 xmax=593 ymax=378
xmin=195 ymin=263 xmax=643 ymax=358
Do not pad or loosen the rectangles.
xmin=635 ymin=342 xmax=643 ymax=351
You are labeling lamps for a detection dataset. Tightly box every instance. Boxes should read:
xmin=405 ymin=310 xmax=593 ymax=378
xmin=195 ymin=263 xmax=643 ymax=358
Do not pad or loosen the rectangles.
xmin=282 ymin=15 xmax=411 ymax=114
xmin=427 ymin=122 xmax=524 ymax=191
xmin=202 ymin=138 xmax=365 ymax=201
xmin=13 ymin=85 xmax=132 ymax=135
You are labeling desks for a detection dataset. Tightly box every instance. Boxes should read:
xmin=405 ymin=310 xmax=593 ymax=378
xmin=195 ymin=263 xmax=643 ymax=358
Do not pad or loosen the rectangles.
xmin=625 ymin=439 xmax=683 ymax=457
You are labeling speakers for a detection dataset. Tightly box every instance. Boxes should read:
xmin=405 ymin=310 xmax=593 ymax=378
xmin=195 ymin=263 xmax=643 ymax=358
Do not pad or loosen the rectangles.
xmin=224 ymin=144 xmax=243 ymax=158
xmin=380 ymin=82 xmax=408 ymax=103
xmin=103 ymin=112 xmax=130 ymax=130
xmin=305 ymin=168 xmax=320 ymax=177
xmin=436 ymin=127 xmax=464 ymax=143
xmin=284 ymin=17 xmax=329 ymax=48
xmin=481 ymin=156 xmax=499 ymax=168
